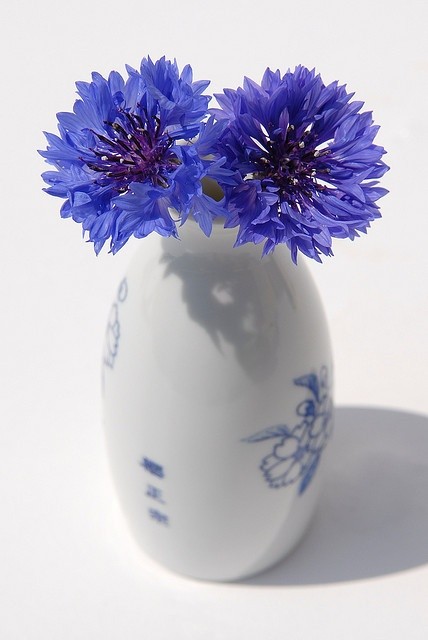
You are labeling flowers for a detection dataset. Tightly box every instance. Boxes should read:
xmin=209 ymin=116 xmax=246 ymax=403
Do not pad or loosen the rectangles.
xmin=35 ymin=53 xmax=392 ymax=263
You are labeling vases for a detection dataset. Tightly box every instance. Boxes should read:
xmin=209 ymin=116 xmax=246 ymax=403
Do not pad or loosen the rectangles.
xmin=95 ymin=177 xmax=337 ymax=583
